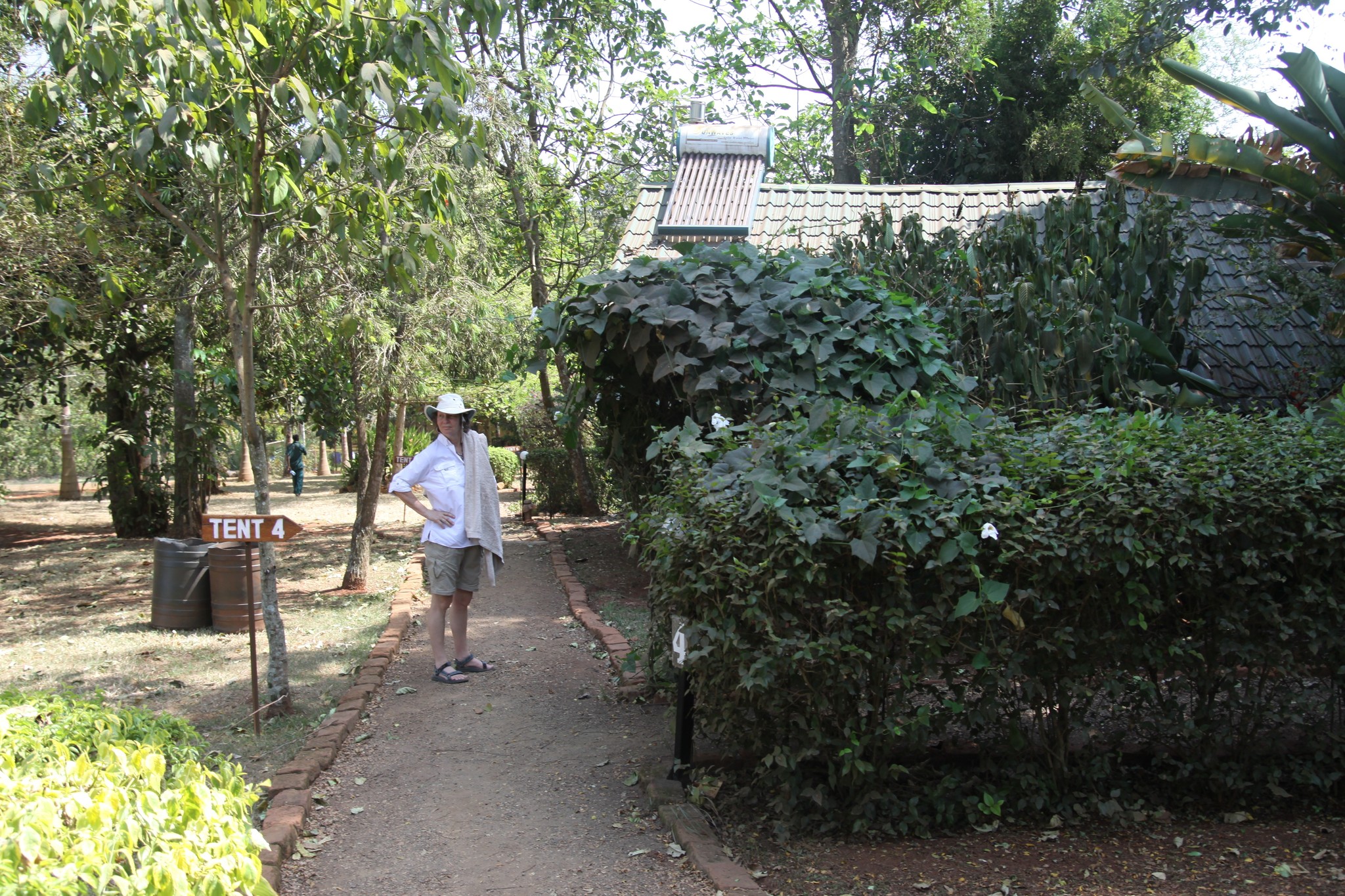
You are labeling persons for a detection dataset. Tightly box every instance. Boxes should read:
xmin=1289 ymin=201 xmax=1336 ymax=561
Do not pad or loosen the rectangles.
xmin=285 ymin=434 xmax=307 ymax=496
xmin=389 ymin=393 xmax=506 ymax=684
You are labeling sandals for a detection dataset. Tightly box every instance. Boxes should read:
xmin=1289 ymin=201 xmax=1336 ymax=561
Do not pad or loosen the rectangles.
xmin=431 ymin=661 xmax=469 ymax=684
xmin=454 ymin=654 xmax=496 ymax=672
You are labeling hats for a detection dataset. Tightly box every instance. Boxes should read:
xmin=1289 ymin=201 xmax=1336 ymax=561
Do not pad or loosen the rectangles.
xmin=423 ymin=393 xmax=477 ymax=421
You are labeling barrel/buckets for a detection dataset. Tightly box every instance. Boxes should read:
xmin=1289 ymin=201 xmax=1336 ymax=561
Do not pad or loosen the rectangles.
xmin=147 ymin=533 xmax=269 ymax=633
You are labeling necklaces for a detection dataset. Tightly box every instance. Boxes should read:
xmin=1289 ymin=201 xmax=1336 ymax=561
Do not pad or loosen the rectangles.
xmin=456 ymin=450 xmax=464 ymax=460
